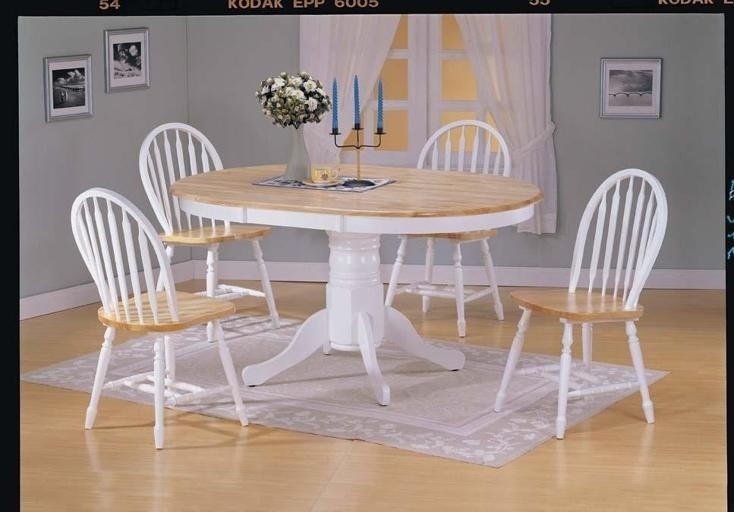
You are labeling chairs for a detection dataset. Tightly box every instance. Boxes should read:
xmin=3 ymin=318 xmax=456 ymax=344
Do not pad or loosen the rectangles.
xmin=493 ymin=167 xmax=669 ymax=441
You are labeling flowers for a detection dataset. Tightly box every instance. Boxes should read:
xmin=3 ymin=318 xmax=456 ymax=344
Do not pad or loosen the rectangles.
xmin=254 ymin=72 xmax=332 ymax=131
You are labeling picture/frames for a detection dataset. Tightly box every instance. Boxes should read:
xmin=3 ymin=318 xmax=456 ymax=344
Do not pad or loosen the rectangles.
xmin=42 ymin=52 xmax=94 ymax=124
xmin=598 ymin=56 xmax=663 ymax=119
xmin=103 ymin=27 xmax=150 ymax=95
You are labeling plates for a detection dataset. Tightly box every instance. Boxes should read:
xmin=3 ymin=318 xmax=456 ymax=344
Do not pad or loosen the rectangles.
xmin=301 ymin=180 xmax=339 ymax=187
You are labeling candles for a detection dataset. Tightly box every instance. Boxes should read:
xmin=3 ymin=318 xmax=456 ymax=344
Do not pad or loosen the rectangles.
xmin=331 ymin=75 xmax=384 ymax=127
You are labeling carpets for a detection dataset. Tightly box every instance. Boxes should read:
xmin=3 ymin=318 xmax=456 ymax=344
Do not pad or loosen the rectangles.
xmin=21 ymin=311 xmax=670 ymax=470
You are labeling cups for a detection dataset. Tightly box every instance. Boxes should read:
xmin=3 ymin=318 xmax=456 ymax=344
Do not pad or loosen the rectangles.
xmin=310 ymin=166 xmax=341 ymax=184
